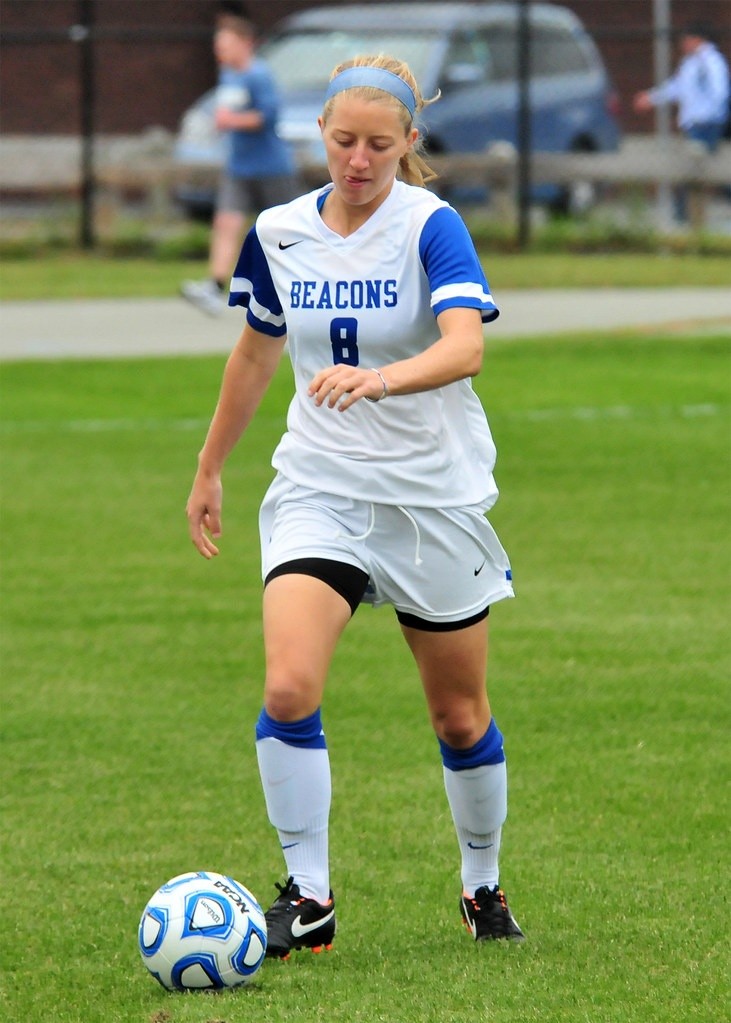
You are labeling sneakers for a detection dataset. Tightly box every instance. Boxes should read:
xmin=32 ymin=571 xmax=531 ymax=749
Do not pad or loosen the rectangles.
xmin=458 ymin=887 xmax=527 ymax=944
xmin=265 ymin=873 xmax=336 ymax=959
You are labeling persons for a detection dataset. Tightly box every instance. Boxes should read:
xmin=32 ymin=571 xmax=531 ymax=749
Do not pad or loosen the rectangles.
xmin=186 ymin=55 xmax=525 ymax=961
xmin=632 ymin=21 xmax=731 ymax=230
xmin=179 ymin=15 xmax=299 ymax=319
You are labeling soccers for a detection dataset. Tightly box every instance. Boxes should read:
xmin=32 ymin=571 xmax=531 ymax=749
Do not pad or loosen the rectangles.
xmin=137 ymin=869 xmax=267 ymax=995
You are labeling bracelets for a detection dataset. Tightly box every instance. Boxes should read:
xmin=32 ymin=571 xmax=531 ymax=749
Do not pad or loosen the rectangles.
xmin=365 ymin=368 xmax=388 ymax=403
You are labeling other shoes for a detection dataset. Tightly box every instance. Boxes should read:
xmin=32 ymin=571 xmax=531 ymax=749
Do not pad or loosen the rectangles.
xmin=179 ymin=280 xmax=228 ymax=317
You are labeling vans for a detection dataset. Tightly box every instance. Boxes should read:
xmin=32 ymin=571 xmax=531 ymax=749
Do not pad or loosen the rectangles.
xmin=167 ymin=1 xmax=618 ymax=239
